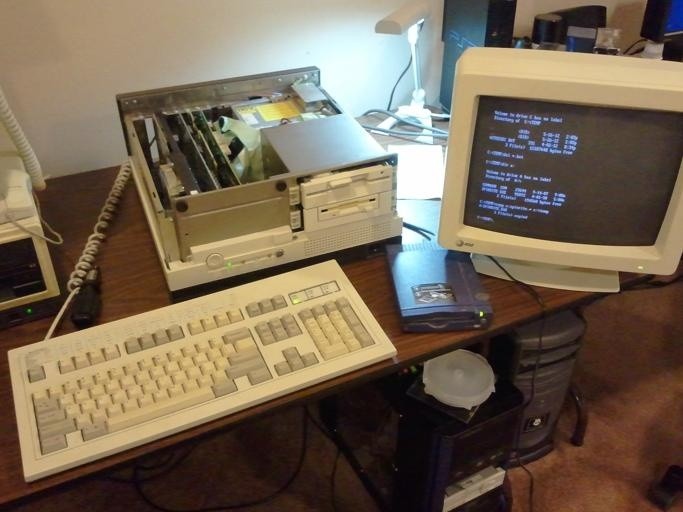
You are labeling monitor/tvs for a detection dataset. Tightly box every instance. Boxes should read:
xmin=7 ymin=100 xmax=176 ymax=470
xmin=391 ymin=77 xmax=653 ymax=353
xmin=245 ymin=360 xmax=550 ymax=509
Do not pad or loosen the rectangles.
xmin=639 ymin=0 xmax=683 ymax=44
xmin=436 ymin=46 xmax=683 ymax=293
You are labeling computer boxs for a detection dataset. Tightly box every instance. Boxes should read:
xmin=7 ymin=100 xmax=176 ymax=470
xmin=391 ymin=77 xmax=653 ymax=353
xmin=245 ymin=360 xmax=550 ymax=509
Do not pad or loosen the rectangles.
xmin=324 ymin=344 xmax=527 ymax=511
xmin=113 ymin=64 xmax=403 ymax=291
xmin=497 ymin=308 xmax=587 ymax=469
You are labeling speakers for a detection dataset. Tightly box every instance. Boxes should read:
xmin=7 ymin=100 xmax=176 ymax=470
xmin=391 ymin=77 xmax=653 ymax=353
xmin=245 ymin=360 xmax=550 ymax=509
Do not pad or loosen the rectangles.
xmin=436 ymin=0 xmax=518 ymax=116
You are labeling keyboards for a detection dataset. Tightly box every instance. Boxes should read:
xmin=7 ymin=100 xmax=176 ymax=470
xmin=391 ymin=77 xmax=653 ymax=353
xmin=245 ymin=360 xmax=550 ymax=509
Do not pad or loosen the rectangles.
xmin=6 ymin=257 xmax=400 ymax=484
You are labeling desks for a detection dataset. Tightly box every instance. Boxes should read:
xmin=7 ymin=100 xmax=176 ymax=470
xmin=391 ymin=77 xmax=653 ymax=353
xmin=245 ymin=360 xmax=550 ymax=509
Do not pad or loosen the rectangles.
xmin=1 ymin=103 xmax=623 ymax=512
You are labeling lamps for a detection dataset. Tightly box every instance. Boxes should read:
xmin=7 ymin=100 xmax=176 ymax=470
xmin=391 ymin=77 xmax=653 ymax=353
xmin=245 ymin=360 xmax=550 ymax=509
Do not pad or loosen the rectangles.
xmin=373 ymin=0 xmax=436 ymax=145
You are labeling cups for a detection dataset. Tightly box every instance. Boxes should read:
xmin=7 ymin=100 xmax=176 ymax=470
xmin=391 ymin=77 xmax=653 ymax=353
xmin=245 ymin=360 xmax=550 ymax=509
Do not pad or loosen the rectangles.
xmin=566 ymin=26 xmax=597 ymax=52
xmin=594 ymin=27 xmax=625 ymax=56
